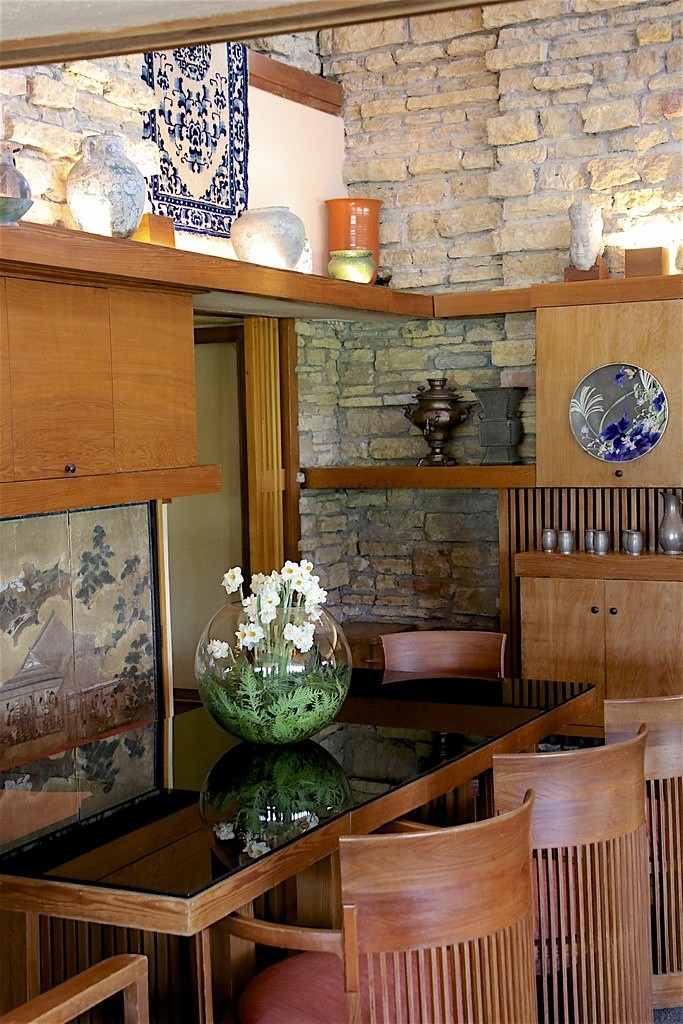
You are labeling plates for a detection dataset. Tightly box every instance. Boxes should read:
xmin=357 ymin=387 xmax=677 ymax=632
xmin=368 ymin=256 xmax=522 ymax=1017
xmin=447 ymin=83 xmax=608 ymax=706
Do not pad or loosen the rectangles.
xmin=568 ymin=362 xmax=668 ymax=463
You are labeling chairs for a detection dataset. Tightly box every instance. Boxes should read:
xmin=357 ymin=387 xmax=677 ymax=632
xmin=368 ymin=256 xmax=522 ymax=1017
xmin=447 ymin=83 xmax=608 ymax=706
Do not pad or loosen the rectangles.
xmin=0 ymin=631 xmax=683 ymax=1024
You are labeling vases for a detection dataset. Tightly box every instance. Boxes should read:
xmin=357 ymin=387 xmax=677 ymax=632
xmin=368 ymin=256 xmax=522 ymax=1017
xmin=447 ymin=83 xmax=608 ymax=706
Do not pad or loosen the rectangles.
xmin=230 ymin=206 xmax=306 ymax=269
xmin=199 ymin=739 xmax=354 ymax=870
xmin=194 ymin=600 xmax=352 ymax=744
xmin=65 ymin=135 xmax=147 ymax=239
xmin=325 ymin=199 xmax=384 ymax=284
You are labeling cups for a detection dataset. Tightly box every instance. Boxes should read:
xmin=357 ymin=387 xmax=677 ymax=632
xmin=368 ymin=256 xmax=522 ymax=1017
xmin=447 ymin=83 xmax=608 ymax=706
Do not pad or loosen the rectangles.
xmin=585 ymin=529 xmax=595 ymax=553
xmin=558 ymin=531 xmax=573 ymax=555
xmin=594 ymin=531 xmax=608 ymax=554
xmin=629 ymin=532 xmax=643 ymax=556
xmin=542 ymin=529 xmax=557 ymax=552
xmin=622 ymin=531 xmax=632 ymax=553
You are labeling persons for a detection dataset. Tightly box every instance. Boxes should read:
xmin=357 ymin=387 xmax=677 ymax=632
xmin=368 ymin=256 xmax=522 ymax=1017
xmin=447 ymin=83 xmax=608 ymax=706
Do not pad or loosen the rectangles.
xmin=568 ymin=201 xmax=604 ymax=271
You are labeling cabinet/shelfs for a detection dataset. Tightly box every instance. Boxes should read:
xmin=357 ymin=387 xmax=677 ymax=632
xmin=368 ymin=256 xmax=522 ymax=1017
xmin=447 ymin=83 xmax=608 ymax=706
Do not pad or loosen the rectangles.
xmin=0 ymin=277 xmax=116 ymax=482
xmin=515 ymin=551 xmax=683 ymax=737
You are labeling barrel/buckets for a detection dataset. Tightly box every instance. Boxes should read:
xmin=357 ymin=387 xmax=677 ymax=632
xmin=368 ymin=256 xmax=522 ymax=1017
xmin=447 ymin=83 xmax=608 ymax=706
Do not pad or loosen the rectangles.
xmin=325 ymin=198 xmax=384 ymax=284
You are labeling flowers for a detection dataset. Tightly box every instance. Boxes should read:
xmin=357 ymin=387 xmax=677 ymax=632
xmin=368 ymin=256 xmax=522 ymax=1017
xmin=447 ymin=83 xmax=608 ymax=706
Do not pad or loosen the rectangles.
xmin=203 ymin=748 xmax=349 ymax=859
xmin=199 ymin=559 xmax=349 ymax=741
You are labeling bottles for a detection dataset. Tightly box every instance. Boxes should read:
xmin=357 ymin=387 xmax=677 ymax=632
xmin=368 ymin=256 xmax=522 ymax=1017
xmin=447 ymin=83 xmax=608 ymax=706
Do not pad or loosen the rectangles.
xmin=328 ymin=249 xmax=376 ymax=284
xmin=65 ymin=134 xmax=147 ymax=239
xmin=0 ymin=152 xmax=34 ymax=228
xmin=230 ymin=207 xmax=306 ymax=270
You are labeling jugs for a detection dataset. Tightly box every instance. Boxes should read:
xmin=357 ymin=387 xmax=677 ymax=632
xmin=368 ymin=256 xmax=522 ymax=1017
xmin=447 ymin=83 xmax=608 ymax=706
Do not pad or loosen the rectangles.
xmin=657 ymin=491 xmax=683 ymax=555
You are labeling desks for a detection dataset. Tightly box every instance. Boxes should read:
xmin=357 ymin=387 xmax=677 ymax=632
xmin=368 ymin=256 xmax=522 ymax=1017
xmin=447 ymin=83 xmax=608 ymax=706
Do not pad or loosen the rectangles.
xmin=0 ymin=668 xmax=598 ymax=1024
